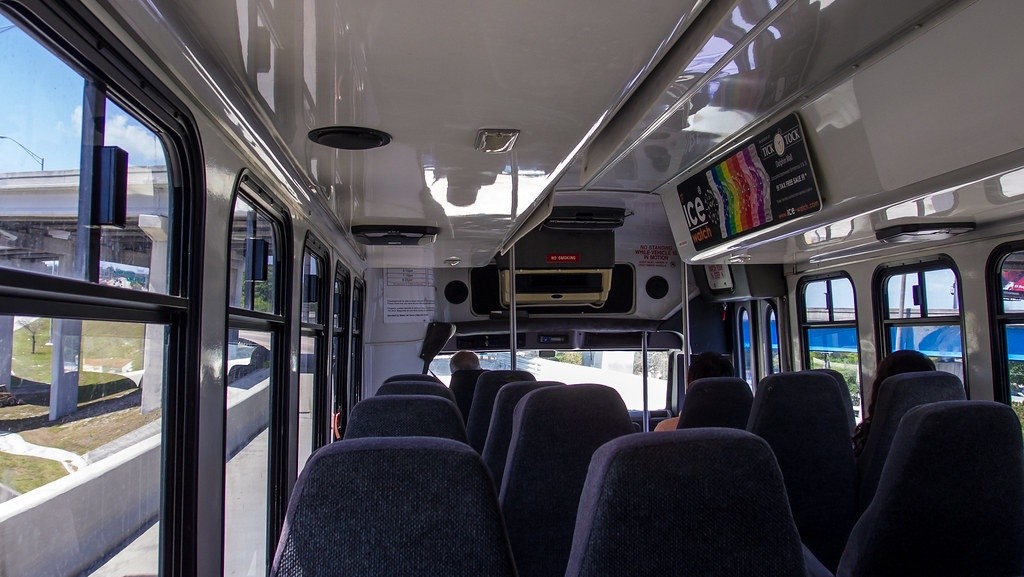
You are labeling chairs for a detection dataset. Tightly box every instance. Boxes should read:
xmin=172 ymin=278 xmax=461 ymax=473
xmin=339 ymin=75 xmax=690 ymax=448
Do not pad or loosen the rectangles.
xmin=269 ymin=367 xmax=1024 ymax=577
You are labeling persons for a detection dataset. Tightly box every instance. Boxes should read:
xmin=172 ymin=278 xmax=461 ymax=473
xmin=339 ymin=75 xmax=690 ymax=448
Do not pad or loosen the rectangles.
xmin=652 ymin=352 xmax=735 ymax=432
xmin=853 ymin=349 xmax=936 ymax=458
xmin=449 ymin=350 xmax=482 ymax=374
